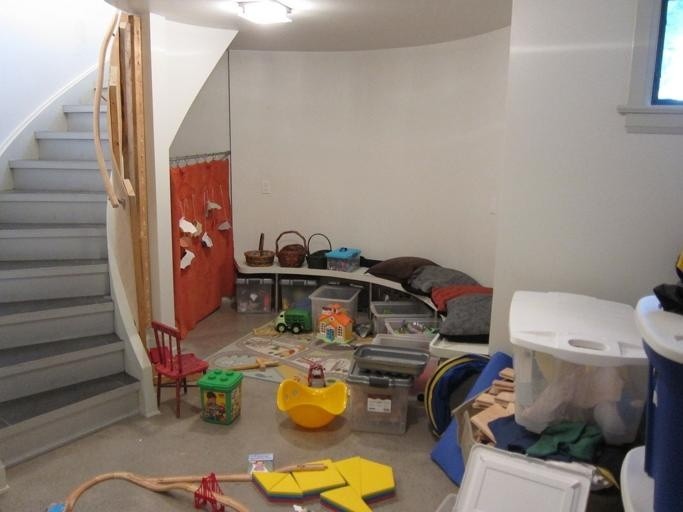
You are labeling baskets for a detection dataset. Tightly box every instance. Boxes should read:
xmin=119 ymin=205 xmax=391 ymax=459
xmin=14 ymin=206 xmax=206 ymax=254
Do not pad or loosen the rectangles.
xmin=244 ymin=233 xmax=274 ymax=266
xmin=306 ymin=232 xmax=331 ymax=269
xmin=275 ymin=230 xmax=306 ymax=268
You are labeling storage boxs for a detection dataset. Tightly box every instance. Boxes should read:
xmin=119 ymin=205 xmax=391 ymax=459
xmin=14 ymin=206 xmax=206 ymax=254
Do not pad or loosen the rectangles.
xmin=231 ymin=275 xmax=441 ymax=435
xmin=506 ymin=287 xmax=682 ymax=511
xmin=324 ymin=246 xmax=361 ymax=272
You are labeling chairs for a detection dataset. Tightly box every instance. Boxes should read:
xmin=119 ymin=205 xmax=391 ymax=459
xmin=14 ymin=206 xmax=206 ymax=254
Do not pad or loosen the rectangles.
xmin=150 ymin=320 xmax=208 ymax=418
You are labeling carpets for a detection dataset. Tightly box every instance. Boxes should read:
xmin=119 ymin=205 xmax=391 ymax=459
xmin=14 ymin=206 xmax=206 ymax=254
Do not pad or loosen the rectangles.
xmin=201 ymin=311 xmax=372 ymax=397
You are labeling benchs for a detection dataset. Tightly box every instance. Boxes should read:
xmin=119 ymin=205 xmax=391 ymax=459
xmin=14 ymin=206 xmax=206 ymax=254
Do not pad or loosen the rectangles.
xmin=235 ymin=258 xmax=490 ymax=366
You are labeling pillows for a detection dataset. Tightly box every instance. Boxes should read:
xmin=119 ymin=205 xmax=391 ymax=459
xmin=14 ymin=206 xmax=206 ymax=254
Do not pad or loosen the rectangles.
xmin=365 ymin=254 xmax=493 ymax=342
